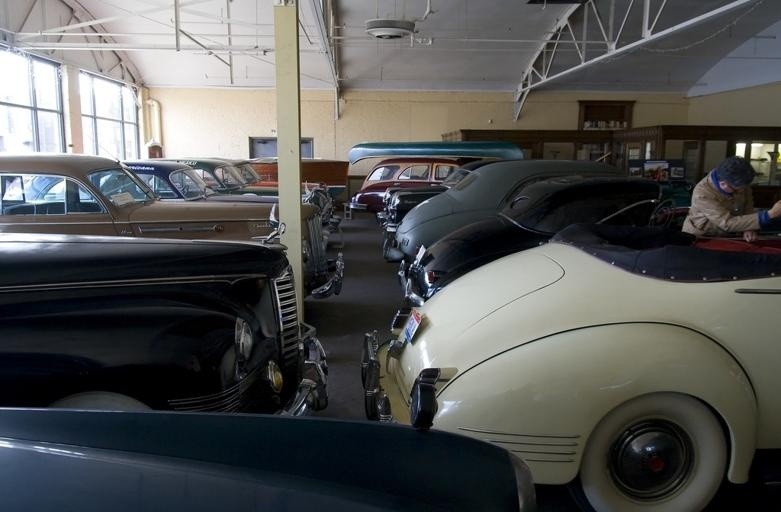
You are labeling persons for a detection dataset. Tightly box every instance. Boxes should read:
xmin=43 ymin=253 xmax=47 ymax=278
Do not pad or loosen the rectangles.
xmin=681 ymin=156 xmax=781 ymax=244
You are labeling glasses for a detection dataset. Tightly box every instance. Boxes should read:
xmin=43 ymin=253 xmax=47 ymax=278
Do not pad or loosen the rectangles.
xmin=726 ymin=183 xmax=751 ymax=195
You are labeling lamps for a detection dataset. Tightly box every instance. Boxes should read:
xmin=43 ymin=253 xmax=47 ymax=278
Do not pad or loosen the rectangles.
xmin=365 ymin=1 xmax=415 ymax=40
